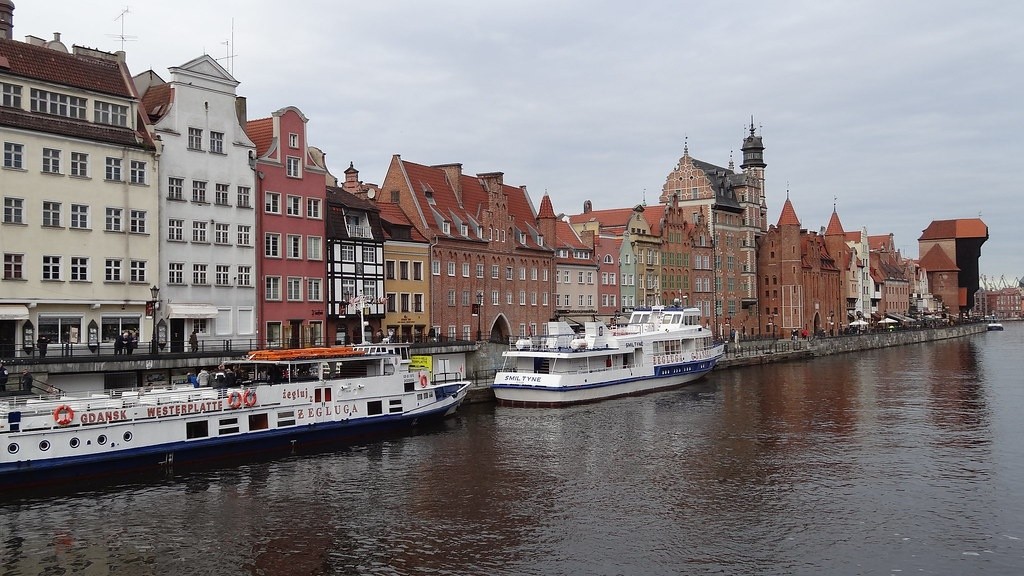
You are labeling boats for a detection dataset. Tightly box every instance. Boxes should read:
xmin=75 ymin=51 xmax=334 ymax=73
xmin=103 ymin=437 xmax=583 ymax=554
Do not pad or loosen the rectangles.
xmin=491 ymin=296 xmax=725 ymax=403
xmin=988 ymin=323 xmax=1004 ymax=331
xmin=0 ymin=341 xmax=471 ymax=476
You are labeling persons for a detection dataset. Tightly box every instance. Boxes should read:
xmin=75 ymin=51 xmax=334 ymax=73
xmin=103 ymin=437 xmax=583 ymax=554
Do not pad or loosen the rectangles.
xmin=114 ymin=335 xmax=124 ymax=355
xmin=428 ymin=326 xmax=436 ymax=343
xmin=818 ymin=327 xmax=857 ymax=340
xmin=126 ymin=333 xmax=134 ymax=354
xmin=790 ymin=328 xmax=807 ymax=340
xmin=267 ymin=364 xmax=283 ymax=380
xmin=730 ymin=329 xmax=735 ymax=342
xmin=208 ymin=365 xmax=249 ymax=388
xmin=373 ymin=329 xmax=397 ymax=343
xmin=37 ymin=336 xmax=50 ymax=358
xmin=344 ymin=333 xmax=350 ymax=344
xmin=196 ymin=367 xmax=209 ymax=387
xmin=21 ymin=369 xmax=33 ymax=391
xmin=189 ymin=331 xmax=198 ymax=351
xmin=297 ymin=366 xmax=315 ymax=380
xmin=0 ymin=362 xmax=9 ymax=392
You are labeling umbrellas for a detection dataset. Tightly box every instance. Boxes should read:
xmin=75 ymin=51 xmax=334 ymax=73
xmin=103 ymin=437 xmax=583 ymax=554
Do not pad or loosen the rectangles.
xmin=878 ymin=318 xmax=898 ymax=329
xmin=849 ymin=320 xmax=869 ymax=334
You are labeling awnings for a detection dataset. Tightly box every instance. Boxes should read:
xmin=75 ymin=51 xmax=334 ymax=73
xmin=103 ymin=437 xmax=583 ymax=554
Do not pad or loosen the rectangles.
xmin=0 ymin=305 xmax=30 ymax=320
xmin=165 ymin=303 xmax=218 ymax=318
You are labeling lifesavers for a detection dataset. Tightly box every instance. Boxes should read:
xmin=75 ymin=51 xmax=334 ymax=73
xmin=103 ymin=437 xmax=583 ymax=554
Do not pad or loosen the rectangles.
xmin=243 ymin=390 xmax=257 ymax=408
xmin=53 ymin=405 xmax=74 ymax=425
xmin=228 ymin=391 xmax=242 ymax=409
xmin=420 ymin=375 xmax=428 ymax=388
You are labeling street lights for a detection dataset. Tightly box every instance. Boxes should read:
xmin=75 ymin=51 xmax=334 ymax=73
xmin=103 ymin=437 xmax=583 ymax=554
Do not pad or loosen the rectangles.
xmin=150 ymin=285 xmax=160 ymax=353
xmin=475 ymin=293 xmax=483 ymax=342
